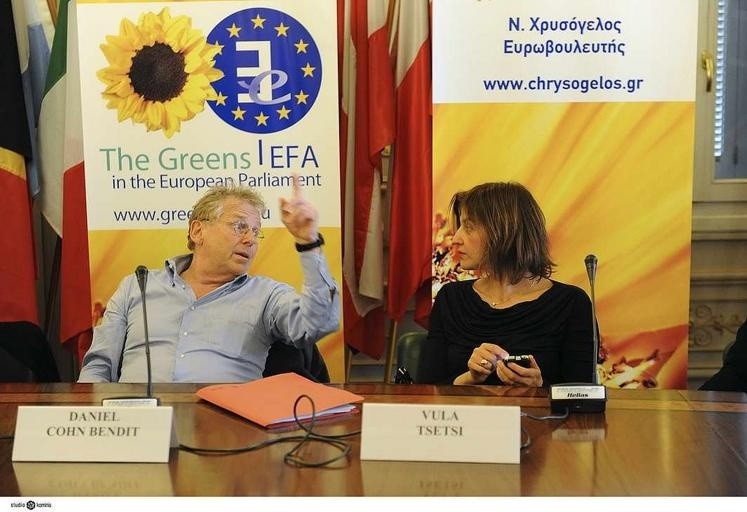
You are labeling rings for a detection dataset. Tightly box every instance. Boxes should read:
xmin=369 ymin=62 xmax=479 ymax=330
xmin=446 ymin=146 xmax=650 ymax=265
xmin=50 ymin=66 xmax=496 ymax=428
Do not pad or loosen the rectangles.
xmin=510 ymin=381 xmax=517 ymax=387
xmin=479 ymin=358 xmax=489 ymax=365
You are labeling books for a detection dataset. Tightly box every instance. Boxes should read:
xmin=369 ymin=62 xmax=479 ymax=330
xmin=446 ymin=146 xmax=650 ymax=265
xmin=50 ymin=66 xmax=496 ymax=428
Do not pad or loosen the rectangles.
xmin=194 ymin=371 xmax=367 ymax=429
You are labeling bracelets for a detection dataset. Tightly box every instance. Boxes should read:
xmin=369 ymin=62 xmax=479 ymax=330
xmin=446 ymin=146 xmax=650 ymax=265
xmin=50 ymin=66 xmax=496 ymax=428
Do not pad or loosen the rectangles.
xmin=294 ymin=231 xmax=326 ymax=253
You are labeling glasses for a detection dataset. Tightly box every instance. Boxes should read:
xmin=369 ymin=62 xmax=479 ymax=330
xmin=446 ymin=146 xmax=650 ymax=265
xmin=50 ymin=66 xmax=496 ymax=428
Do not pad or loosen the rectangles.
xmin=198 ymin=216 xmax=266 ymax=247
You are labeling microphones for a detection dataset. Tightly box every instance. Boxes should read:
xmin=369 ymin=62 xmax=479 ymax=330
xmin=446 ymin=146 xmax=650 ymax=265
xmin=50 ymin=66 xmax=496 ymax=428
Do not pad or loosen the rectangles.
xmin=101 ymin=265 xmax=160 ymax=409
xmin=549 ymin=253 xmax=607 ymax=414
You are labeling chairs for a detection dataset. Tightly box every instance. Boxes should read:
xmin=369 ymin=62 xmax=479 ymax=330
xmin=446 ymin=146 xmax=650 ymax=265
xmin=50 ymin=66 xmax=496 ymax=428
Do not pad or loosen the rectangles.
xmin=393 ymin=330 xmax=428 ymax=384
xmin=264 ymin=334 xmax=331 ymax=385
xmin=0 ymin=320 xmax=62 ymax=383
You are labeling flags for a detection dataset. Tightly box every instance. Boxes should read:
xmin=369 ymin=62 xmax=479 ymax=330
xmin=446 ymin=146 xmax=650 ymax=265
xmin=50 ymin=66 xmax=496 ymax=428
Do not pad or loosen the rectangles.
xmin=0 ymin=0 xmax=64 ymax=388
xmin=35 ymin=0 xmax=94 ymax=372
xmin=386 ymin=2 xmax=435 ymax=333
xmin=337 ymin=1 xmax=396 ymax=362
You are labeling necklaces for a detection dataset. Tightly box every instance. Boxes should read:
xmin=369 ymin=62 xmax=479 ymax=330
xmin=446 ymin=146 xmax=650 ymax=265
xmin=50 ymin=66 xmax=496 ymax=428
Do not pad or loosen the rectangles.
xmin=478 ymin=279 xmax=512 ymax=308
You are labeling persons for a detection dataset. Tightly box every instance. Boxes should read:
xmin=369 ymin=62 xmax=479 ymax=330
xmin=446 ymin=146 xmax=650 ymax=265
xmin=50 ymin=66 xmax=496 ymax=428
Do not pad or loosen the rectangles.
xmin=411 ymin=181 xmax=599 ymax=390
xmin=697 ymin=321 xmax=746 ymax=394
xmin=75 ymin=166 xmax=343 ymax=384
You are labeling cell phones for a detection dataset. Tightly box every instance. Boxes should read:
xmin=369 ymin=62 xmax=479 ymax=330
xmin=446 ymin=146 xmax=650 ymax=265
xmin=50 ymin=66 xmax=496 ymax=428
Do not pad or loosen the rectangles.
xmin=503 ymin=355 xmax=530 ymax=368
